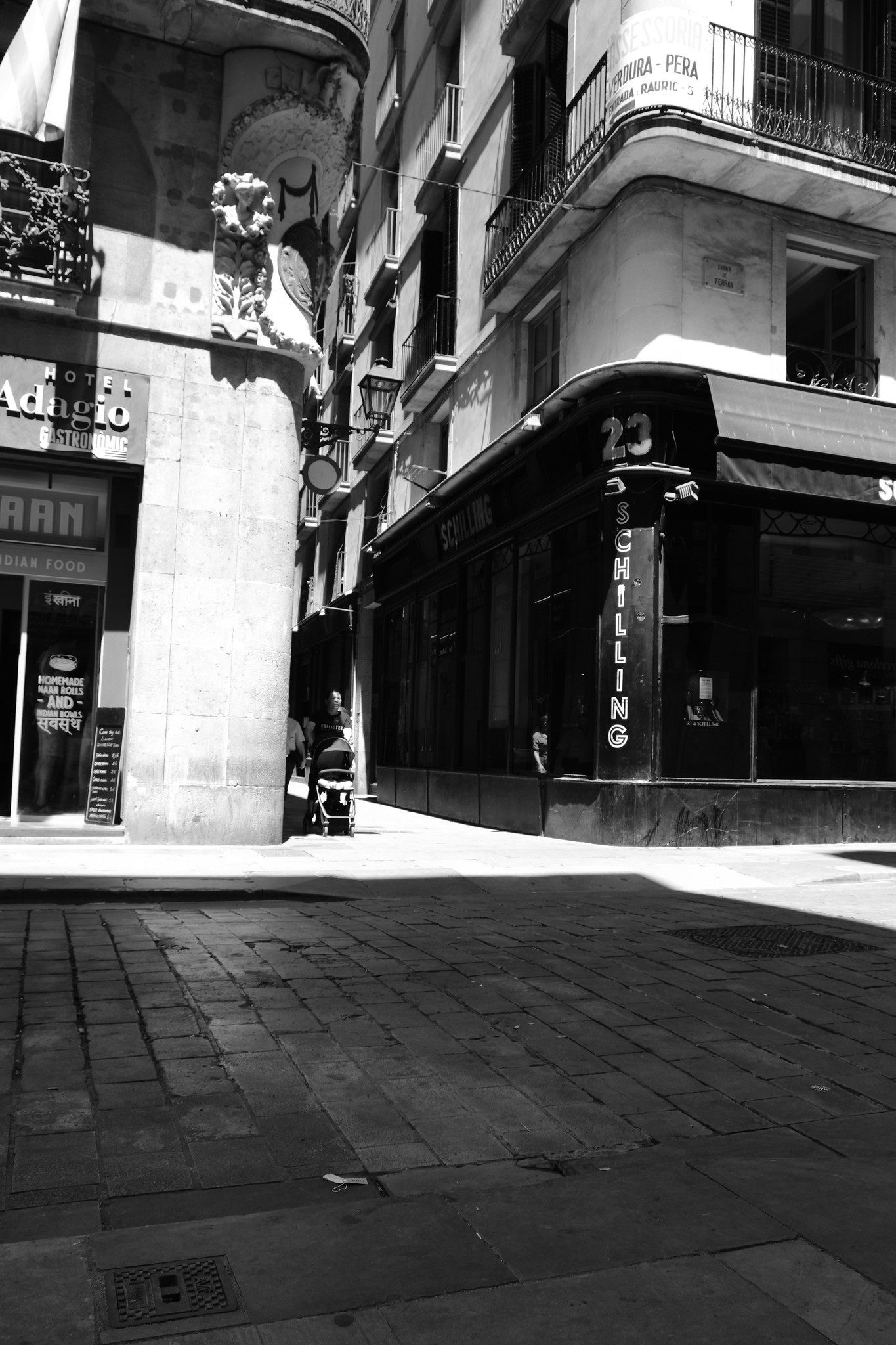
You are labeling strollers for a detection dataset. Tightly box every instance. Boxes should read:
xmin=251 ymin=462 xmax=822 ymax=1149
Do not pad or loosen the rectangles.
xmin=311 ymin=737 xmax=357 ymax=838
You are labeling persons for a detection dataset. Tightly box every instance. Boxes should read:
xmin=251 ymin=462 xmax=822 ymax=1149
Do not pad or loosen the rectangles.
xmin=224 ymin=181 xmax=262 ymax=228
xmin=532 ymin=714 xmax=549 ymax=777
xmin=283 ymin=703 xmax=307 ymax=813
xmin=302 ymin=689 xmax=353 ymax=834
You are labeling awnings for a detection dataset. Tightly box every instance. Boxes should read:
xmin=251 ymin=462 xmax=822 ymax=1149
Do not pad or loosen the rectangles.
xmin=702 ymin=374 xmax=896 ymax=509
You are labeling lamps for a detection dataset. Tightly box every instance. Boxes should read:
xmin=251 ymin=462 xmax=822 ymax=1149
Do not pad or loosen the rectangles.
xmin=521 ymin=413 xmax=541 ymax=433
xmin=424 ymin=497 xmax=440 ymax=510
xmin=301 ymin=354 xmax=404 ymax=457
xmin=367 ymin=544 xmax=379 ymax=554
xmin=664 ymin=480 xmax=703 ymax=505
